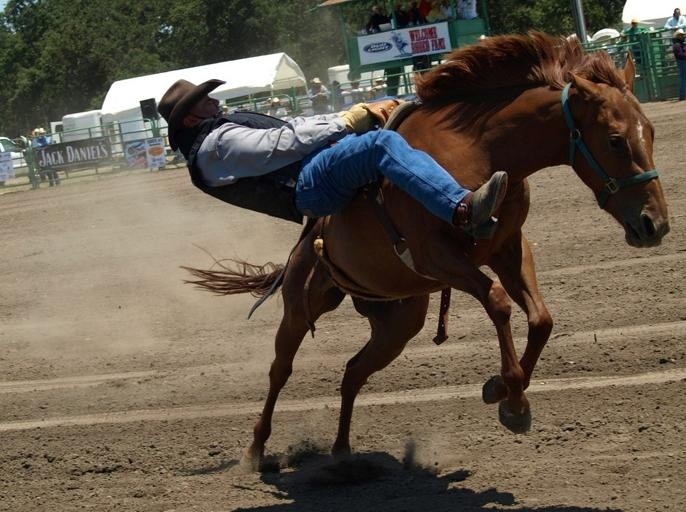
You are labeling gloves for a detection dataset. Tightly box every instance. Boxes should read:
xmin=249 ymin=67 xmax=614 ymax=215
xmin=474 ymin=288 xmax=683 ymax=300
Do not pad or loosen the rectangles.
xmin=341 ymin=99 xmax=379 ymax=136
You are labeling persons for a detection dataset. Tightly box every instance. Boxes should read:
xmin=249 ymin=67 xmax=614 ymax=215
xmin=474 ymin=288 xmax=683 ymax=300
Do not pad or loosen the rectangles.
xmin=365 ymin=0 xmax=476 ymax=35
xmin=31 ymin=128 xmax=60 ymax=188
xmin=664 ymin=8 xmax=686 ymax=35
xmin=155 ymin=78 xmax=508 ymax=245
xmin=672 ymin=29 xmax=686 ymax=100
xmin=618 ymin=16 xmax=655 ymax=64
xmin=307 ymin=78 xmax=330 ymax=114
xmin=368 ymin=78 xmax=389 ymax=100
xmin=330 ymin=80 xmax=347 ymax=113
xmin=270 ymin=97 xmax=283 ymax=118
xmin=351 ymin=77 xmax=363 ymax=103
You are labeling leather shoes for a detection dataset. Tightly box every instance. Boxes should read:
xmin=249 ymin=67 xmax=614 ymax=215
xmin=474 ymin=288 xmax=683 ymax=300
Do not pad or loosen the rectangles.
xmin=452 ymin=171 xmax=511 ymax=241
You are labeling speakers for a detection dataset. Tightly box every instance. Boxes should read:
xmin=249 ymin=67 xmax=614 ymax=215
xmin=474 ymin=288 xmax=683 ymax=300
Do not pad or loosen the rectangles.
xmin=140 ymin=98 xmax=159 ymax=122
xmin=412 ymin=55 xmax=431 ymax=73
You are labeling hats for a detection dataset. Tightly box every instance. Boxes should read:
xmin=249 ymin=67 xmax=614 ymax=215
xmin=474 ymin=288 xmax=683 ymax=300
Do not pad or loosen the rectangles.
xmin=630 ymin=17 xmax=641 ymax=25
xmin=672 ymin=28 xmax=686 ymax=38
xmin=154 ymin=78 xmax=226 ymax=153
xmin=34 ymin=128 xmax=47 ymax=134
xmin=271 ymin=97 xmax=279 ymax=103
xmin=374 ymin=76 xmax=384 ymax=82
xmin=309 ymin=78 xmax=322 ymax=84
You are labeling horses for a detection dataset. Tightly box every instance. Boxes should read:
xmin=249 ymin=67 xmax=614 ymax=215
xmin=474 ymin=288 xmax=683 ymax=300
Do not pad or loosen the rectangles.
xmin=177 ymin=29 xmax=670 ymax=476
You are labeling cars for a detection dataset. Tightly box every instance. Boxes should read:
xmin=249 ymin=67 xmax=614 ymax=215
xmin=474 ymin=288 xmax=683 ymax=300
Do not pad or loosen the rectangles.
xmin=0 ymin=137 xmax=28 ymax=176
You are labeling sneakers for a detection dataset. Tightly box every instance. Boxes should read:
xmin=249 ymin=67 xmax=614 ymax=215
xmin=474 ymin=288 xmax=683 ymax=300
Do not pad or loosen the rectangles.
xmin=48 ymin=181 xmax=61 ymax=187
